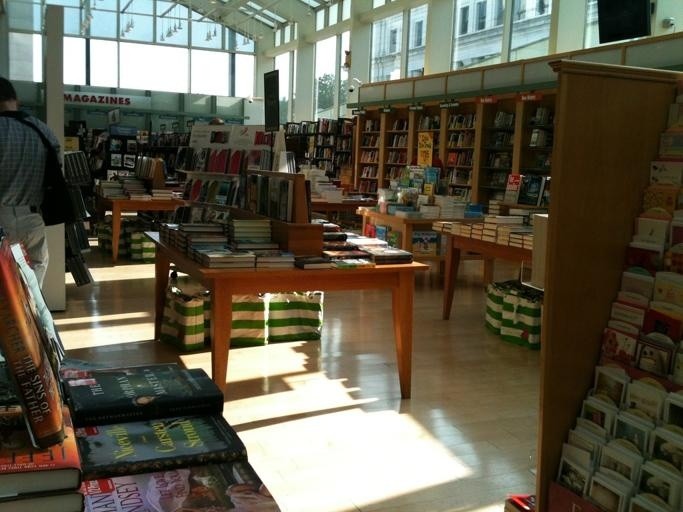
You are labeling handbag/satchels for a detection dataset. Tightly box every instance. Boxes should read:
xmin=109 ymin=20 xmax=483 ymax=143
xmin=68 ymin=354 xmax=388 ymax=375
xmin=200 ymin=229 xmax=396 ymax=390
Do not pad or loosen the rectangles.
xmin=42 ymin=148 xmax=76 ymax=226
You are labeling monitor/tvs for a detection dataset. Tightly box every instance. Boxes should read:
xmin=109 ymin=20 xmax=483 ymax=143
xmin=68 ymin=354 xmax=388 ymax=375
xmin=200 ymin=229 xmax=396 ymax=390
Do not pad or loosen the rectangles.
xmin=596 ymin=0 xmax=658 ymax=45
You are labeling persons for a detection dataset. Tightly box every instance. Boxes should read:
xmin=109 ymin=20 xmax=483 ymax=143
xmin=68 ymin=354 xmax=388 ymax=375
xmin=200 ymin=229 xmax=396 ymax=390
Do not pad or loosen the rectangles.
xmin=0 ymin=75 xmax=66 ymax=292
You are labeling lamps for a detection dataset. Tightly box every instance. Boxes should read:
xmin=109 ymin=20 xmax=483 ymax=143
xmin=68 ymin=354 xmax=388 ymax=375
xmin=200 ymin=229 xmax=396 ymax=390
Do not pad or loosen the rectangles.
xmin=78 ymin=0 xmax=315 ymax=45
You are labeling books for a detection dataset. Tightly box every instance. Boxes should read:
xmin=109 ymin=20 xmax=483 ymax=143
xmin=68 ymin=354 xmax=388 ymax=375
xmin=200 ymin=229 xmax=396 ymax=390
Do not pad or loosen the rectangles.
xmin=295 ymin=210 xmax=416 ymax=271
xmin=558 ymin=86 xmax=682 ymax=510
xmin=504 ymin=494 xmax=536 ymax=512
xmin=98 ymin=153 xmax=182 ymax=201
xmin=284 ymin=90 xmax=355 ymax=206
xmin=1 ymin=234 xmax=284 ymax=512
xmin=481 ymin=101 xmax=556 ymax=207
xmin=158 ymin=212 xmax=295 ymax=269
xmin=357 ymin=105 xmax=478 ymax=219
xmin=434 ymin=199 xmax=533 ymax=249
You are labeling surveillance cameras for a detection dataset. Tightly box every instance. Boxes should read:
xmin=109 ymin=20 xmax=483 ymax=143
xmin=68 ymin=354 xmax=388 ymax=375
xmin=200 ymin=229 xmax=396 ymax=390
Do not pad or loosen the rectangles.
xmin=348 ymin=85 xmax=355 ymax=93
xmin=248 ymin=98 xmax=254 ymax=106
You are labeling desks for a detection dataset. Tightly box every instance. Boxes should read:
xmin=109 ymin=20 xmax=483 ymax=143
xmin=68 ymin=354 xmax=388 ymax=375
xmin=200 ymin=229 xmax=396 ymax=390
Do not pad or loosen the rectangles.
xmin=58 ymin=338 xmax=195 ymax=369
xmin=96 ymin=197 xmax=187 ymax=265
xmin=311 ymin=201 xmax=377 ymax=227
xmin=437 ymin=229 xmax=531 ymax=323
xmin=353 ymin=208 xmax=493 ymax=289
xmin=143 ymin=229 xmax=431 ymax=399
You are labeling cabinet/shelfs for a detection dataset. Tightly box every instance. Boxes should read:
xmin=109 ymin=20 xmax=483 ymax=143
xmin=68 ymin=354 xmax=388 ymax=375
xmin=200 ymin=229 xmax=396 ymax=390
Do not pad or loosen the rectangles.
xmin=284 ymin=117 xmax=354 ymax=180
xmin=534 ymin=55 xmax=683 ymax=512
xmin=173 ymin=124 xmax=285 ymax=226
xmin=353 ymin=88 xmax=554 ymax=208
